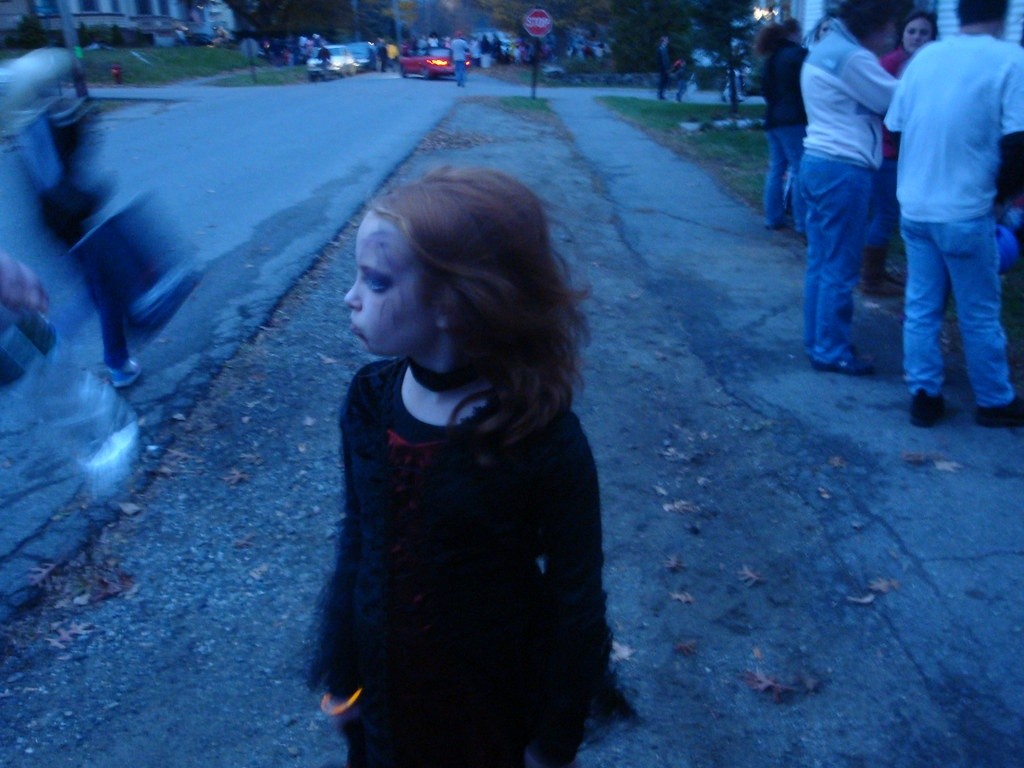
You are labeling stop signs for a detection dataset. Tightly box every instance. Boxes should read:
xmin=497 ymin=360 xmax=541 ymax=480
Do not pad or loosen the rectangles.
xmin=523 ymin=10 xmax=553 ymax=38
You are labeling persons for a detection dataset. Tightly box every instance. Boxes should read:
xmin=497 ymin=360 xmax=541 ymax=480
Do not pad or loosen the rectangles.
xmin=303 ymin=171 xmax=640 ymax=768
xmin=672 ymin=59 xmax=688 ymax=102
xmin=258 ymin=31 xmax=609 ymax=89
xmin=657 ymin=36 xmax=670 ymax=99
xmin=753 ymin=0 xmax=1024 ymax=428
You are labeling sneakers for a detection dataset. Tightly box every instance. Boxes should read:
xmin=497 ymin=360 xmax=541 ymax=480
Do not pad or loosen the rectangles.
xmin=811 ymin=354 xmax=876 ymax=376
xmin=976 ymin=396 xmax=1024 ymax=428
xmin=910 ymin=389 xmax=945 ymax=427
xmin=109 ymin=360 xmax=142 ymax=388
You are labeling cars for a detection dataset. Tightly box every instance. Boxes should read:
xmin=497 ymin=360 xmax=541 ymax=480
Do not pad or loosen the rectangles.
xmin=307 ymin=44 xmax=357 ymax=82
xmin=346 ymin=41 xmax=376 ymax=73
xmin=399 ymin=47 xmax=471 ymax=80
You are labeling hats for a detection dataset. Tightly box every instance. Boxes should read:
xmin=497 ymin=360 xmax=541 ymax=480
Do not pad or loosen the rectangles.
xmin=456 ymin=31 xmax=463 ymax=37
